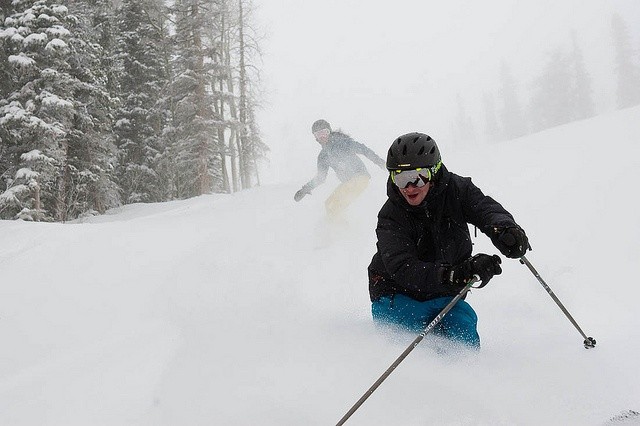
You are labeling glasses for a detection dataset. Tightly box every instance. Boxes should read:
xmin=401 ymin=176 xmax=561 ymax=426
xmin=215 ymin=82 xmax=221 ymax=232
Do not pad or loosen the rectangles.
xmin=313 ymin=128 xmax=330 ymax=138
xmin=391 ymin=160 xmax=443 ymax=190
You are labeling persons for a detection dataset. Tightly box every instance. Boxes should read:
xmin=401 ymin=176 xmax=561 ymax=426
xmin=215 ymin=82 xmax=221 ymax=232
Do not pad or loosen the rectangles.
xmin=295 ymin=116 xmax=386 ymax=228
xmin=367 ymin=132 xmax=532 ymax=347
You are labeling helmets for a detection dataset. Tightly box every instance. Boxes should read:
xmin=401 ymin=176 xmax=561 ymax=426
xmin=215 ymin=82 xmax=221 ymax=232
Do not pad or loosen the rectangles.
xmin=386 ymin=132 xmax=441 ymax=185
xmin=312 ymin=120 xmax=331 ymax=134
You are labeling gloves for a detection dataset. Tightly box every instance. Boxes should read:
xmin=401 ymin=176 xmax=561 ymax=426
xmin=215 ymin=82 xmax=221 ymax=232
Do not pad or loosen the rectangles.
xmin=294 ymin=186 xmax=309 ymax=201
xmin=448 ymin=254 xmax=502 ymax=287
xmin=485 ymin=221 xmax=532 ymax=259
